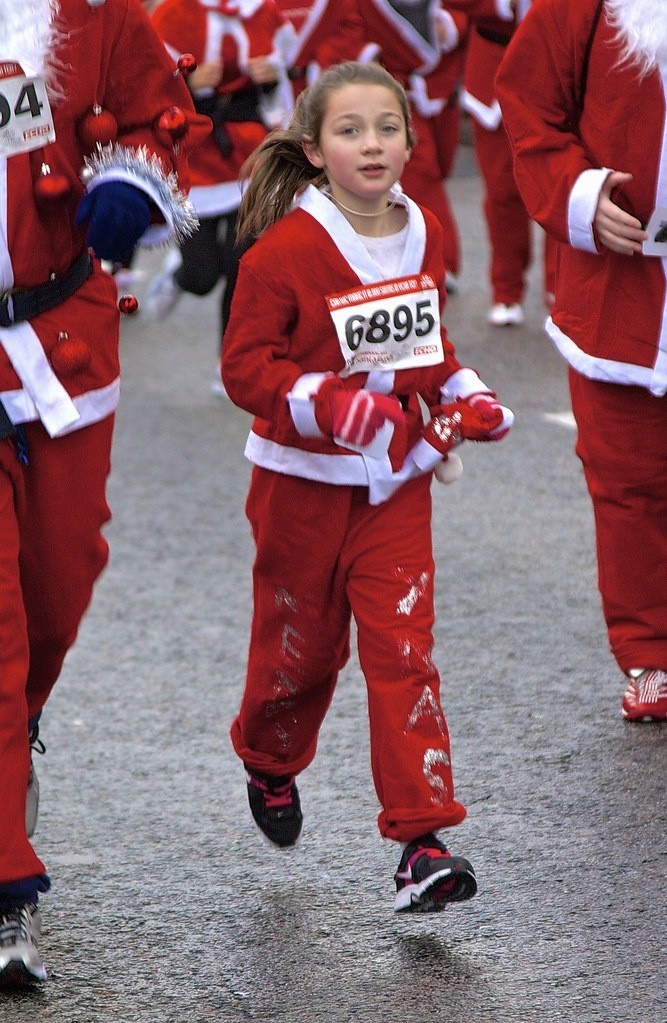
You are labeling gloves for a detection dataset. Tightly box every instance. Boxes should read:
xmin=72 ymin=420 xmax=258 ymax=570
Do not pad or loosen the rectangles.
xmin=465 ymin=394 xmax=514 ymax=441
xmin=310 ymin=377 xmax=405 ymax=447
xmin=75 ymin=181 xmax=150 ymax=268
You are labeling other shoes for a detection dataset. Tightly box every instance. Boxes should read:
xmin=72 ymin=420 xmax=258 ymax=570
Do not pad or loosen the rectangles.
xmin=443 ymin=271 xmax=457 ymax=293
xmin=144 ymin=248 xmax=182 ymax=321
xmin=211 ymin=365 xmax=227 ymax=395
xmin=489 ymin=302 xmax=525 ymax=325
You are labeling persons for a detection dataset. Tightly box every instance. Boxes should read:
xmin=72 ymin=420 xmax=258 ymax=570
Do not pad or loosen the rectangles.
xmin=111 ymin=0 xmax=558 ymax=329
xmin=0 ymin=0 xmax=216 ymax=987
xmin=495 ymin=0 xmax=667 ymax=724
xmin=218 ymin=62 xmax=513 ymax=913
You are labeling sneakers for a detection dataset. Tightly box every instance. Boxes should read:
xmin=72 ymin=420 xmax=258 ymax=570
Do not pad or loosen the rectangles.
xmin=243 ymin=762 xmax=303 ymax=848
xmin=24 ymin=707 xmax=47 ymax=839
xmin=621 ymin=668 xmax=667 ymax=722
xmin=394 ymin=832 xmax=477 ymax=915
xmin=0 ymin=901 xmax=47 ymax=989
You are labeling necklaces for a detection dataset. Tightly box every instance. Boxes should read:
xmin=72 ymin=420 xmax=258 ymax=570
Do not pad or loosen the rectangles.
xmin=324 ymin=190 xmax=401 ymax=217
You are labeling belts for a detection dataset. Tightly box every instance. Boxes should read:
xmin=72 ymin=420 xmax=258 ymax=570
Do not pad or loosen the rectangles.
xmin=475 ymin=24 xmax=511 ymax=46
xmin=193 ymin=86 xmax=264 ymax=157
xmin=0 ymin=252 xmax=92 ymax=328
